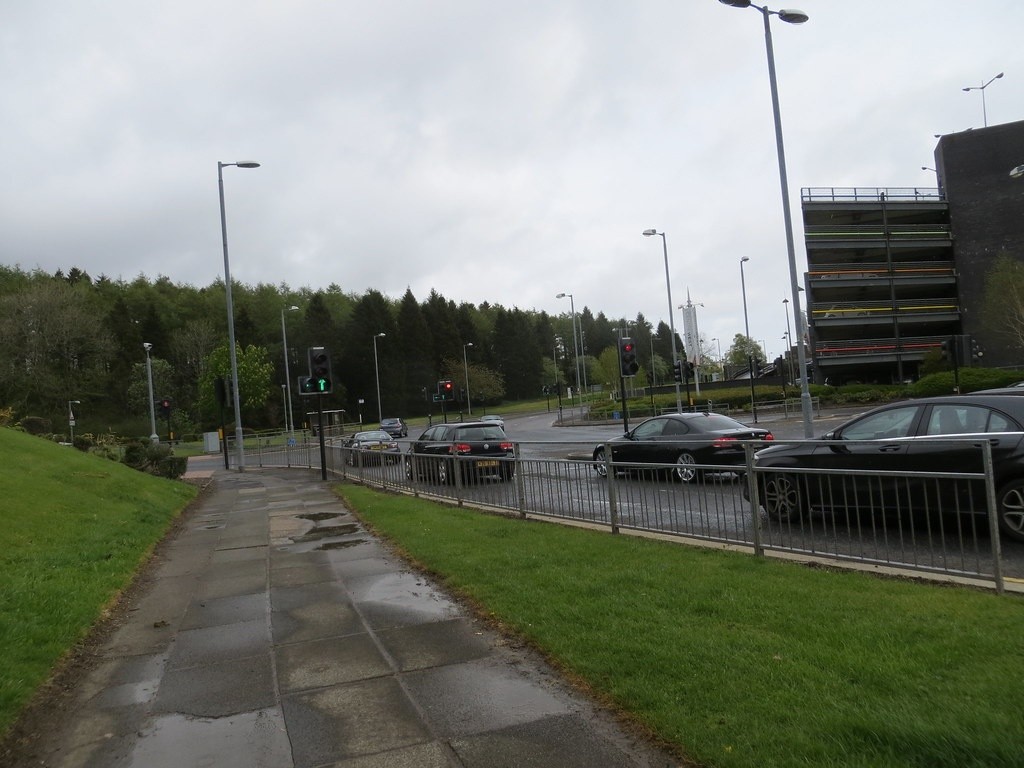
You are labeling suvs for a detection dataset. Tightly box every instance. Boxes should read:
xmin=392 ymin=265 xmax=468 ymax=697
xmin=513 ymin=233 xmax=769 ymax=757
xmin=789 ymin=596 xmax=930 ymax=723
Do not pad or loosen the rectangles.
xmin=405 ymin=422 xmax=516 ymax=486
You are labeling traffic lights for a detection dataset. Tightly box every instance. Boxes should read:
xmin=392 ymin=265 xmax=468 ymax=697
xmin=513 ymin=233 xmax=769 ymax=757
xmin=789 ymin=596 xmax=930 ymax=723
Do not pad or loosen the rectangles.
xmin=162 ymin=400 xmax=169 ymax=407
xmin=298 ymin=376 xmax=333 ymax=395
xmin=445 ymin=381 xmax=454 ymax=401
xmin=619 ymin=337 xmax=639 ymax=377
xmin=672 ymin=360 xmax=684 ymax=384
xmin=684 ymin=362 xmax=694 ymax=379
xmin=433 ymin=394 xmax=445 ymax=402
xmin=308 ymin=347 xmax=332 ymax=376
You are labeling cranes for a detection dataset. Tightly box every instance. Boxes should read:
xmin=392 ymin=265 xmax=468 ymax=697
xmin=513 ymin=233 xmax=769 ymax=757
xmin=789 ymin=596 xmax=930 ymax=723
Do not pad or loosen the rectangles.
xmin=678 ymin=287 xmax=705 ymax=310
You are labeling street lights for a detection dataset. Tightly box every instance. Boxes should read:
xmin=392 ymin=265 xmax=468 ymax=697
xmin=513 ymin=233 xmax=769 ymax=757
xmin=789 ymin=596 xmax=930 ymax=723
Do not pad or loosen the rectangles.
xmin=721 ymin=1 xmax=822 ymax=441
xmin=644 ymin=227 xmax=685 ymax=413
xmin=740 ymin=255 xmax=759 ymax=423
xmin=463 ymin=342 xmax=473 ymax=416
xmin=143 ymin=342 xmax=160 ymax=446
xmin=612 ymin=319 xmax=662 ymax=385
xmin=218 ymin=157 xmax=261 ymax=471
xmin=69 ymin=401 xmax=80 ymax=443
xmin=374 ymin=332 xmax=386 ymax=416
xmin=712 ymin=338 xmax=720 ymax=362
xmin=556 ymin=293 xmax=588 ymax=421
xmin=358 ymin=399 xmax=365 ymax=432
xmin=780 ymin=298 xmax=796 ymax=385
xmin=281 ymin=306 xmax=300 ymax=439
xmin=962 ymin=72 xmax=1004 ymax=129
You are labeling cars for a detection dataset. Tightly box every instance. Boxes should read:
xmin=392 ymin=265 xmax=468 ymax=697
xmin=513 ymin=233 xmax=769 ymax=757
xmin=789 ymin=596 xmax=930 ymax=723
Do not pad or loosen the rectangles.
xmin=478 ymin=415 xmax=505 ymax=432
xmin=342 ymin=431 xmax=401 ymax=467
xmin=379 ymin=418 xmax=408 ymax=438
xmin=593 ymin=411 xmax=774 ymax=484
xmin=743 ymin=379 xmax=1024 ymax=546
